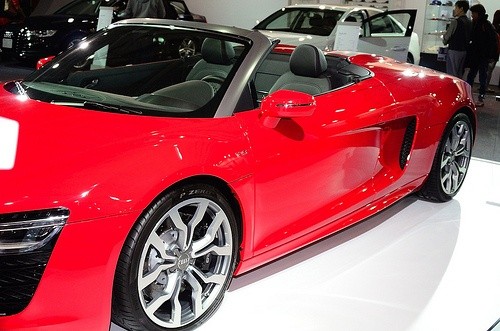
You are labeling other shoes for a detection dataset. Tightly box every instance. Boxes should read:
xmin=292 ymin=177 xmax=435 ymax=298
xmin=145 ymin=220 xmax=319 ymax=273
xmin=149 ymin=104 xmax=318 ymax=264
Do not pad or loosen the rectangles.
xmin=475 ymin=98 xmax=485 ymax=107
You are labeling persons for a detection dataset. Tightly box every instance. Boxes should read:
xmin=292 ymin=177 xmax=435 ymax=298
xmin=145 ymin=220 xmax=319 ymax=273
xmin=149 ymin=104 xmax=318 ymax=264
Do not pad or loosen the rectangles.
xmin=125 ymin=0 xmax=166 ymax=19
xmin=443 ymin=0 xmax=500 ymax=107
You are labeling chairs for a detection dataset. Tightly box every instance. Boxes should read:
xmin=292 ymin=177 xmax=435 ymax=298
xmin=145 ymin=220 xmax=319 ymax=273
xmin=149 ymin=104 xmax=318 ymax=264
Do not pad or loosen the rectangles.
xmin=268 ymin=45 xmax=334 ymax=99
xmin=186 ymin=36 xmax=259 ymax=113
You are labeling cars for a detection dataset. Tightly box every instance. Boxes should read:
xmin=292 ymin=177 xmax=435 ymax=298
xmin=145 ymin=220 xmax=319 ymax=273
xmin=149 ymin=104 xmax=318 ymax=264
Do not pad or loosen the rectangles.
xmin=250 ymin=3 xmax=423 ymax=67
xmin=0 ymin=0 xmax=209 ymax=61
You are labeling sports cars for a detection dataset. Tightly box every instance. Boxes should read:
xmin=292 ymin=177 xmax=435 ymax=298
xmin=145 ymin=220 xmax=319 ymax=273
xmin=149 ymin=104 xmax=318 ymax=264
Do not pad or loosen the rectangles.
xmin=0 ymin=16 xmax=478 ymax=331
